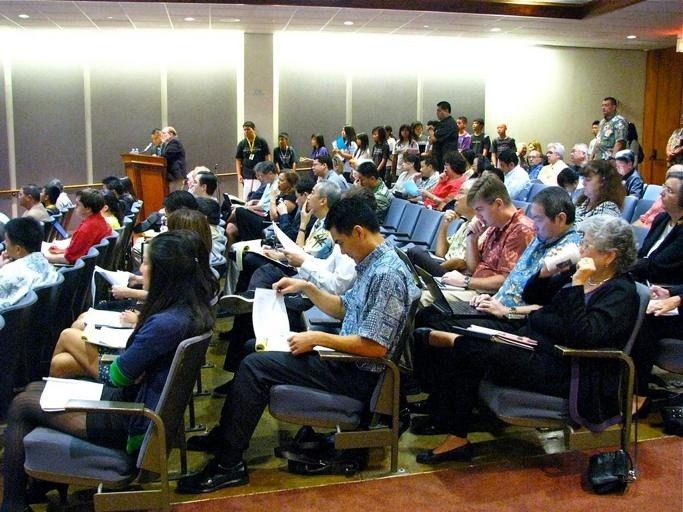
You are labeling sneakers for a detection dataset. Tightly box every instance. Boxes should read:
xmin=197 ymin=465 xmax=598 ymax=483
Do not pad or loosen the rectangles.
xmin=214 ymin=380 xmax=233 ymax=397
xmin=219 ymin=291 xmax=253 ymax=315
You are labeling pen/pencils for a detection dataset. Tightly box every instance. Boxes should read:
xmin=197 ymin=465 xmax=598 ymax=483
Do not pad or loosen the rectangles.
xmin=43 ymin=377 xmax=77 ymax=383
xmin=129 ymin=304 xmax=135 ymax=312
xmin=51 ymin=238 xmax=57 ymax=245
xmin=278 ymin=288 xmax=281 ymax=293
xmin=646 ymin=280 xmax=650 ymax=292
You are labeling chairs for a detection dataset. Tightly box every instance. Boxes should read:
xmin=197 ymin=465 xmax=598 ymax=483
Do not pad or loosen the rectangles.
xmin=268 ymin=287 xmax=421 ymax=475
xmin=40 ymin=205 xmax=77 ymax=243
xmin=23 ymin=331 xmax=213 ymax=510
xmin=207 ymin=211 xmax=231 ymax=317
xmin=509 ymin=177 xmax=662 ymax=250
xmin=378 ymin=198 xmax=463 ymax=254
xmin=1 ymin=257 xmax=86 ymax=425
xmin=476 ymin=282 xmax=651 ymax=453
xmin=77 ymin=198 xmax=149 ymax=313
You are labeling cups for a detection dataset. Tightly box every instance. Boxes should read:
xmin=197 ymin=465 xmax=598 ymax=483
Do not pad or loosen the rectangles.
xmin=543 ymin=242 xmax=582 ymax=274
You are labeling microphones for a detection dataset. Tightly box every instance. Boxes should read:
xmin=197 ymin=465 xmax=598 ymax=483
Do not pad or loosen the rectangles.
xmin=144 ymin=143 xmax=153 ymax=152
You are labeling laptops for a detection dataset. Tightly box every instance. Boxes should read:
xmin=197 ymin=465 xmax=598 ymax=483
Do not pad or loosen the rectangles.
xmin=53 ymin=222 xmax=72 ymax=239
xmin=415 ymin=264 xmax=487 ymax=318
xmin=394 ymin=246 xmax=465 ymax=291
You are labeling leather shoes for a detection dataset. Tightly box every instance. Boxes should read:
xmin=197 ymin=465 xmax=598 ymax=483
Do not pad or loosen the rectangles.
xmin=417 ymin=441 xmax=471 ymax=464
xmin=408 ymin=400 xmax=455 ymax=435
xmin=177 ymin=435 xmax=249 ymax=494
xmin=412 ymin=328 xmax=432 ymax=348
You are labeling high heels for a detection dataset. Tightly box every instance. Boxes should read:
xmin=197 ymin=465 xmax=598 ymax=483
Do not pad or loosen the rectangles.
xmin=26 ymin=483 xmax=68 ymax=502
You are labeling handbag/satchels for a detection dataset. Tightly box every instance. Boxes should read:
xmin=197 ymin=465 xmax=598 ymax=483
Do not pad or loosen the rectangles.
xmin=587 ymin=450 xmax=636 ymax=494
xmin=274 ymin=424 xmax=367 ymax=474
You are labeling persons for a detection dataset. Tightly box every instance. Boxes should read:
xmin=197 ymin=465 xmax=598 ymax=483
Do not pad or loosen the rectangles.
xmin=50 ymin=166 xmax=222 ymax=378
xmin=395 ymin=151 xmax=638 ymax=465
xmin=237 ymin=121 xmax=296 ymax=201
xmin=178 ymin=196 xmax=415 ymax=492
xmin=1 ymin=232 xmax=215 ymax=512
xmin=145 ymin=126 xmax=188 ymax=192
xmin=490 ymin=98 xmax=683 ymax=419
xmin=1 ymin=177 xmax=136 ymax=305
xmin=333 ymin=100 xmax=490 ymax=189
xmin=213 ymin=159 xmax=393 ymax=398
xmin=310 ymin=133 xmax=329 ymax=159
